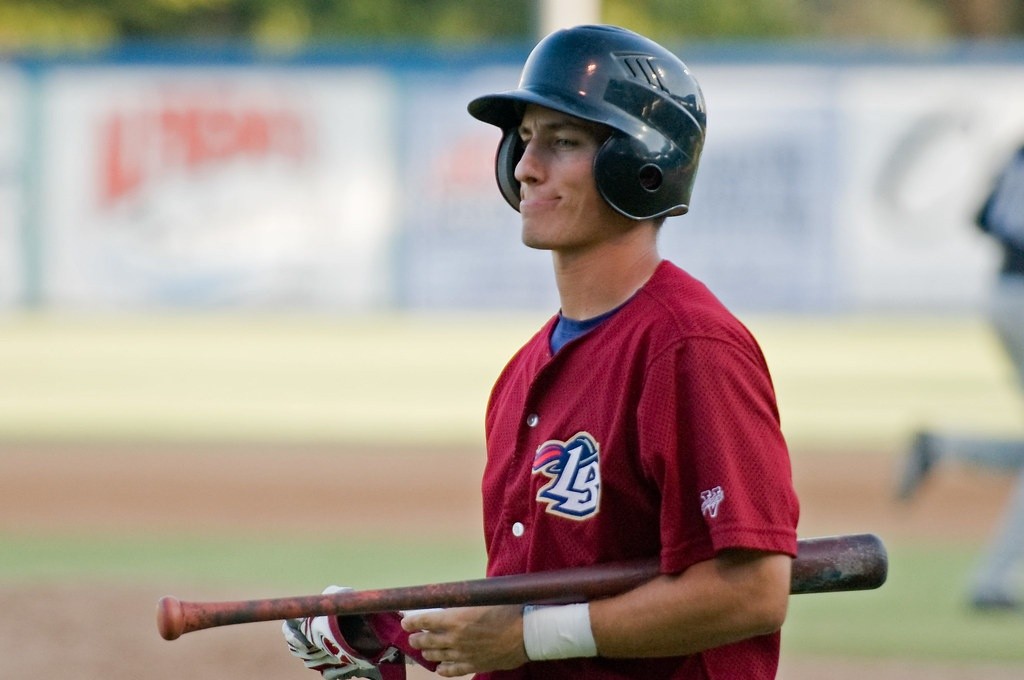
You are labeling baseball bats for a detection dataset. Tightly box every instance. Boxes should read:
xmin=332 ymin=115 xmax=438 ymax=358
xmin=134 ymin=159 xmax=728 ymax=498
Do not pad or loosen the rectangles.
xmin=154 ymin=532 xmax=890 ymax=643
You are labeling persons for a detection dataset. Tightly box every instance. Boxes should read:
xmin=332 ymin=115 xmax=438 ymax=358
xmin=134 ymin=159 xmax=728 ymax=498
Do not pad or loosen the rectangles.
xmin=282 ymin=24 xmax=798 ymax=680
xmin=968 ymin=141 xmax=1024 ymax=605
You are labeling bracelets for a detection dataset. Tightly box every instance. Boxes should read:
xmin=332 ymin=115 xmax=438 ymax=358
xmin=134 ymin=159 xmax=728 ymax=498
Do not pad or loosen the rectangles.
xmin=523 ymin=602 xmax=598 ymax=661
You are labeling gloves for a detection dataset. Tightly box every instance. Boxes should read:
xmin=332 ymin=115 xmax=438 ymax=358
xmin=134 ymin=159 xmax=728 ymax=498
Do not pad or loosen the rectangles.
xmin=281 ymin=586 xmax=443 ymax=680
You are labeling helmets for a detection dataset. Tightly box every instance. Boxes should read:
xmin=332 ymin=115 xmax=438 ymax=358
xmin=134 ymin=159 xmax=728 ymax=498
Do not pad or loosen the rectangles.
xmin=468 ymin=25 xmax=709 ymax=222
